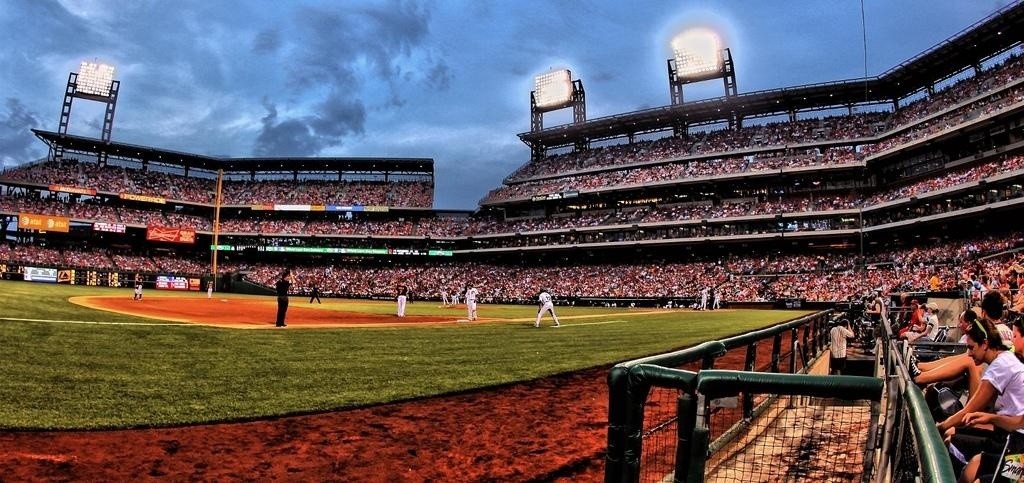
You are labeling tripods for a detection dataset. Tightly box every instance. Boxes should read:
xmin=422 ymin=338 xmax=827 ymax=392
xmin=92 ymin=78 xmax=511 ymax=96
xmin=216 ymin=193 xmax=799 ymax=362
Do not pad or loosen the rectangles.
xmin=847 ymin=319 xmax=873 ymax=355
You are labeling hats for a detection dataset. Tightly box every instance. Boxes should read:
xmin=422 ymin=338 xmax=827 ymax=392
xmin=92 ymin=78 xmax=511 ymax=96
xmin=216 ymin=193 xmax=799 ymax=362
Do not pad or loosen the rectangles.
xmin=925 ymin=302 xmax=939 ymax=310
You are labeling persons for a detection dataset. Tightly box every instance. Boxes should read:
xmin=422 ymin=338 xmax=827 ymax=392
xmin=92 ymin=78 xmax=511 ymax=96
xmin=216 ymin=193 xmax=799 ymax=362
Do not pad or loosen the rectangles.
xmin=1 ymin=157 xmax=434 ymax=304
xmin=434 ymin=53 xmax=1024 ymax=327
xmin=397 ymin=280 xmax=409 ymax=318
xmin=275 ymin=273 xmax=290 ymax=327
xmin=830 ymin=266 xmax=1024 ymax=482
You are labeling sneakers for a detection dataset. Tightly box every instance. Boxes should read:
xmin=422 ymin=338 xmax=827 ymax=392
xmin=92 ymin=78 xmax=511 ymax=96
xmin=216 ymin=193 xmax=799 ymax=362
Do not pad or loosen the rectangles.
xmin=905 ymin=347 xmax=921 ymax=381
xmin=902 ymin=338 xmax=919 ymax=368
